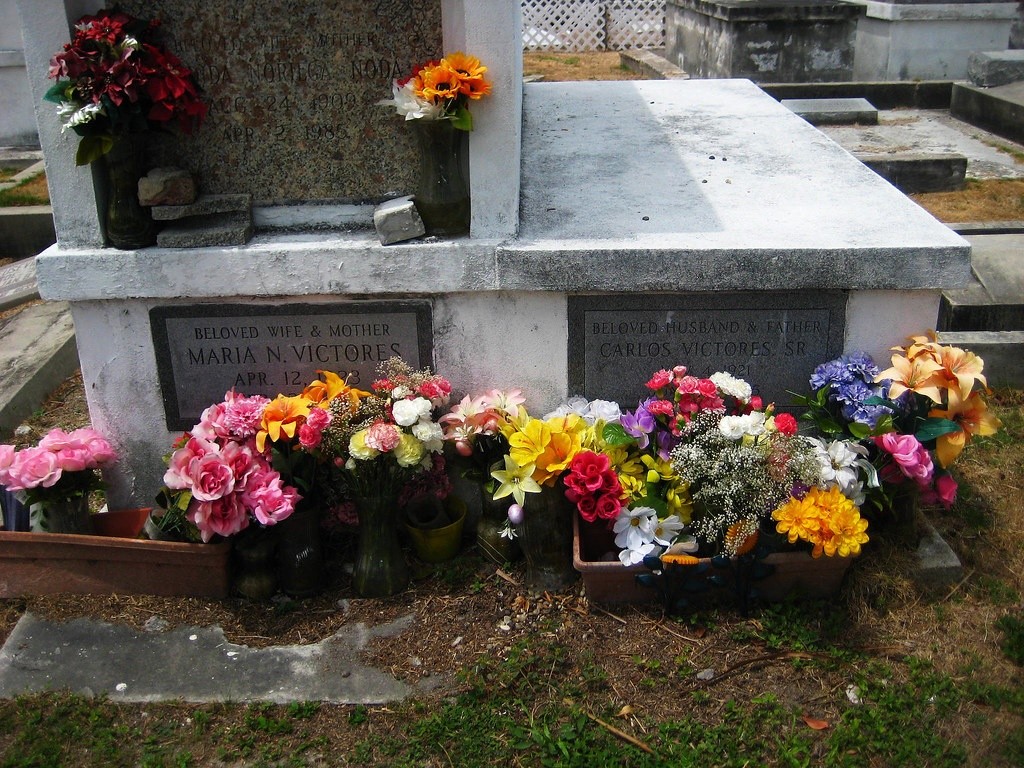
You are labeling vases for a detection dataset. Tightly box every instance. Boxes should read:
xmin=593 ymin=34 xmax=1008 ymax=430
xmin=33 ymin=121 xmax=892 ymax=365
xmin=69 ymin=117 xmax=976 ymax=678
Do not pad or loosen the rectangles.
xmin=226 ymin=529 xmax=282 ymax=604
xmin=475 ymin=489 xmax=524 ymax=565
xmin=44 ymin=487 xmax=96 ymax=537
xmin=570 ymin=503 xmax=854 ymax=603
xmin=412 ymin=121 xmax=471 ymax=234
xmin=515 ymin=484 xmax=573 ymax=586
xmin=349 ymin=491 xmax=410 ymax=598
xmin=401 ymin=497 xmax=467 ymax=562
xmin=102 ymin=149 xmax=159 ymax=250
xmin=138 ymin=507 xmax=185 ymax=542
xmin=268 ymin=496 xmax=322 ymax=597
xmin=0 ymin=484 xmax=30 ymax=532
xmin=871 ymin=485 xmax=914 ymax=524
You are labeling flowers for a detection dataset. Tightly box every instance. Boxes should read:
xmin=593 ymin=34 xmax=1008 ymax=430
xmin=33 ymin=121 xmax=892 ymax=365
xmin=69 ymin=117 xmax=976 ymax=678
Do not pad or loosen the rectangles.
xmin=438 ymin=394 xmax=622 ymax=540
xmin=374 ymin=52 xmax=492 ymax=133
xmin=155 ymin=359 xmax=457 ymax=544
xmin=41 ymin=6 xmax=206 ymax=167
xmin=563 ymin=329 xmax=1002 ymax=567
xmin=0 ymin=428 xmax=116 ymax=503
